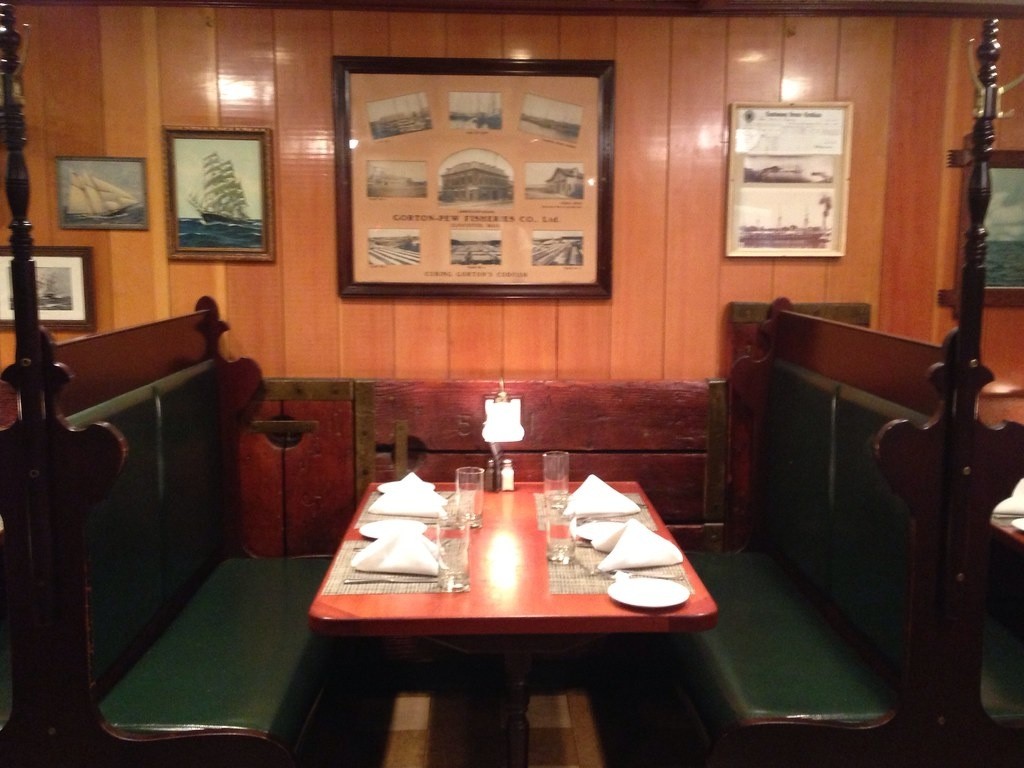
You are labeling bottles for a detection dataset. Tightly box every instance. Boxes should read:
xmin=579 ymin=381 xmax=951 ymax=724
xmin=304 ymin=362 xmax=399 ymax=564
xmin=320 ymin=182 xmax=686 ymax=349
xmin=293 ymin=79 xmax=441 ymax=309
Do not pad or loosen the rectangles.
xmin=501 ymin=459 xmax=515 ymax=491
xmin=485 ymin=459 xmax=495 ymax=492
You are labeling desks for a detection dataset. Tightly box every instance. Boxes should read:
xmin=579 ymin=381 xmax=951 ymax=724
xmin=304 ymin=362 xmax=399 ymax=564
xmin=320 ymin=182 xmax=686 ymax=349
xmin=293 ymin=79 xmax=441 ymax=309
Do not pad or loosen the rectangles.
xmin=305 ymin=476 xmax=719 ymax=705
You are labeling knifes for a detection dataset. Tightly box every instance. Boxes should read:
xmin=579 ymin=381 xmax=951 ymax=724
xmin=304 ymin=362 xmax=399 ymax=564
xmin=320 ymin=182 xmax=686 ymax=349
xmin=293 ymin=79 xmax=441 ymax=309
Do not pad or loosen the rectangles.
xmin=345 ymin=577 xmax=439 ymax=583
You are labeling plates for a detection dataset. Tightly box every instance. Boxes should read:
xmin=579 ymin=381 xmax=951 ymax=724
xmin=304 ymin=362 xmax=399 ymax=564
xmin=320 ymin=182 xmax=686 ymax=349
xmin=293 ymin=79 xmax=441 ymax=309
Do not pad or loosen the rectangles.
xmin=377 ymin=481 xmax=435 ymax=494
xmin=359 ymin=519 xmax=426 ymax=539
xmin=576 ymin=522 xmax=624 ymax=541
xmin=1012 ymin=519 xmax=1024 ymax=530
xmin=608 ymin=579 xmax=690 ymax=608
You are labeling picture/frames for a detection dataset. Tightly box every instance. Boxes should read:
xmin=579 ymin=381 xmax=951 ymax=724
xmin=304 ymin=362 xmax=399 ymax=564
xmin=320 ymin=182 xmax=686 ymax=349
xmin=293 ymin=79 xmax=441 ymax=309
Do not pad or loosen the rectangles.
xmin=328 ymin=51 xmax=616 ymax=300
xmin=935 ymin=143 xmax=1024 ymax=308
xmin=55 ymin=153 xmax=149 ymax=232
xmin=0 ymin=240 xmax=98 ymax=336
xmin=159 ymin=121 xmax=278 ymax=266
xmin=722 ymin=102 xmax=853 ymax=261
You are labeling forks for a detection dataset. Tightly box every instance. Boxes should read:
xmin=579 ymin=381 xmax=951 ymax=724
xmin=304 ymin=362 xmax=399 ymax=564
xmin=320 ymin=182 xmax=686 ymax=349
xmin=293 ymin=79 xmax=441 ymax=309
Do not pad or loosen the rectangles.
xmin=445 ymin=492 xmax=454 ymax=500
xmin=352 ymin=541 xmax=451 ymax=551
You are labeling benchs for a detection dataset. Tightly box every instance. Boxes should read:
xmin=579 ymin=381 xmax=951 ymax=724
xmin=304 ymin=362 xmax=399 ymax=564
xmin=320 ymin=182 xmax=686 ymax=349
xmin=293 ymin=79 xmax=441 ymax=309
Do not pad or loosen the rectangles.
xmin=45 ymin=356 xmax=336 ymax=767
xmin=662 ymin=352 xmax=950 ymax=748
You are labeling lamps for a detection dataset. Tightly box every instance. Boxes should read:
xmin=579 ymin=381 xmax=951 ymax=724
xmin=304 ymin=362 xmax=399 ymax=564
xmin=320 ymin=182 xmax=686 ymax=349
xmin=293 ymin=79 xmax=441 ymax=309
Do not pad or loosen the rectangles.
xmin=482 ymin=393 xmax=526 ymax=492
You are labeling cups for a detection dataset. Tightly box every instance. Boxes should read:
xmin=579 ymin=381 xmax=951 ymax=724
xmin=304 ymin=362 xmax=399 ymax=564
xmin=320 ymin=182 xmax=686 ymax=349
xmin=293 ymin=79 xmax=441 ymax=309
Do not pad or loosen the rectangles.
xmin=455 ymin=466 xmax=485 ymax=529
xmin=437 ymin=516 xmax=470 ymax=592
xmin=543 ymin=451 xmax=569 ymax=507
xmin=547 ymin=495 xmax=576 ymax=566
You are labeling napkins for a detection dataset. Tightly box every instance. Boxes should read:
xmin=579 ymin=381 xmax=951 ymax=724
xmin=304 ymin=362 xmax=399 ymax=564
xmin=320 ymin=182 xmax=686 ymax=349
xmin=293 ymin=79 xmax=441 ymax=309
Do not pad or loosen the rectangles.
xmin=990 ymin=479 xmax=1024 ymax=517
xmin=350 ymin=521 xmax=442 ymax=576
xmin=563 ymin=474 xmax=641 ymax=520
xmin=588 ymin=518 xmax=683 ymax=574
xmin=369 ymin=471 xmax=446 ymax=519
